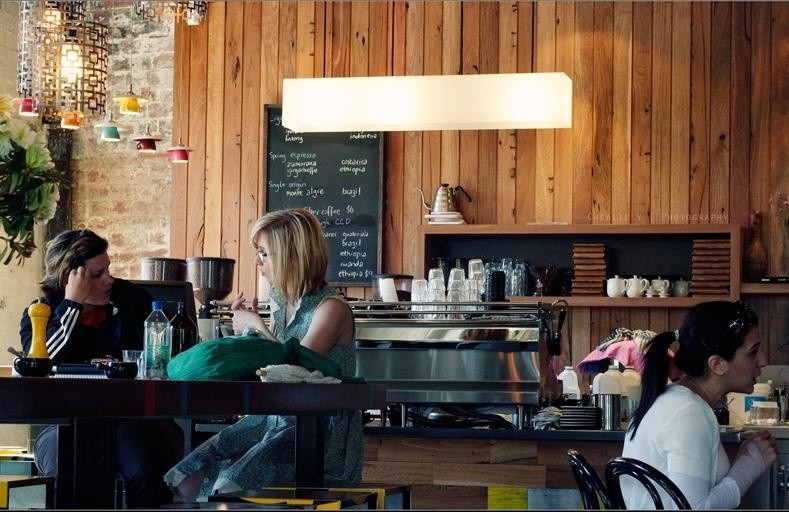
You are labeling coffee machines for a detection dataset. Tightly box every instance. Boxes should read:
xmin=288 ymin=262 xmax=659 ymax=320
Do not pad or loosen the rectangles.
xmin=186 ymin=257 xmax=236 ymax=342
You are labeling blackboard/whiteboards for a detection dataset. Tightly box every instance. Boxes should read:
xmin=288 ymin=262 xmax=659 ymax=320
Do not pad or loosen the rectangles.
xmin=260 ymin=104 xmax=387 ymax=286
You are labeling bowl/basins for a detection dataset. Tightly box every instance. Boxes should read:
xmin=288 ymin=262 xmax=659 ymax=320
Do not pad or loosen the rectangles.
xmin=14 ymin=357 xmax=52 ymax=378
xmin=96 ymin=361 xmax=138 ymax=379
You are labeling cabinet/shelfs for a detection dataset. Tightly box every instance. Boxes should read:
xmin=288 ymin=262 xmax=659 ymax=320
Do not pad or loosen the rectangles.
xmin=417 ymin=223 xmax=788 ymax=307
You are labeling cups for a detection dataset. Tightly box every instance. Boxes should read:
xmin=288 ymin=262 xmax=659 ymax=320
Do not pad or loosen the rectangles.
xmin=591 ymin=394 xmax=621 ymax=431
xmin=409 ymin=257 xmax=486 ymax=320
xmin=607 ymin=274 xmax=692 ymax=298
xmin=484 ymin=253 xmax=529 ymax=297
xmin=559 ymin=406 xmax=603 ymax=430
xmin=123 ymin=349 xmax=143 ymax=380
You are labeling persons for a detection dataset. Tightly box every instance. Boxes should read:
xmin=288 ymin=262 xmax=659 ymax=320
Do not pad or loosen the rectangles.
xmin=163 ymin=207 xmax=364 ymax=506
xmin=618 ymin=300 xmax=778 ymax=510
xmin=19 ymin=229 xmax=185 ymax=510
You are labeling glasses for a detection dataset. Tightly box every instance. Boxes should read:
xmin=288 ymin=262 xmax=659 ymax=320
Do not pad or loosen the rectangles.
xmin=255 ymin=248 xmax=272 ymax=264
xmin=705 ymin=300 xmax=754 ymax=349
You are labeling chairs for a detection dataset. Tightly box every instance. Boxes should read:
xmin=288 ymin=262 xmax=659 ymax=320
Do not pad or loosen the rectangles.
xmin=567 ymin=448 xmax=691 ymax=512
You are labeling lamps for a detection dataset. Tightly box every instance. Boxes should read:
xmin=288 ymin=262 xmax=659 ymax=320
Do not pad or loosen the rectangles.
xmin=280 ymin=0 xmax=573 ymax=133
xmin=13 ymin=0 xmax=194 ymax=163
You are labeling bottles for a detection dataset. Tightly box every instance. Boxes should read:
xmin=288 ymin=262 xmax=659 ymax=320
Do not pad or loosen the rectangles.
xmin=169 ymin=302 xmax=198 ymax=358
xmin=144 ymin=301 xmax=171 ymax=380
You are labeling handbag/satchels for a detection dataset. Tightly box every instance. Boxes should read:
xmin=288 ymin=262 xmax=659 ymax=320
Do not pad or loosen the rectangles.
xmin=166 ymin=336 xmax=341 ymax=381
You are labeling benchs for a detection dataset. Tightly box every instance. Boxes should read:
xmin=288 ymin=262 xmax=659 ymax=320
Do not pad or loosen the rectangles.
xmin=0 ymin=474 xmax=58 ymax=511
xmin=209 ymin=478 xmax=414 ymax=511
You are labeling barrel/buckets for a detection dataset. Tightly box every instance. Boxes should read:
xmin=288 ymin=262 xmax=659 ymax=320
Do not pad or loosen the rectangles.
xmin=592 ymin=365 xmax=641 ymax=401
xmin=727 ymin=383 xmax=769 ymax=426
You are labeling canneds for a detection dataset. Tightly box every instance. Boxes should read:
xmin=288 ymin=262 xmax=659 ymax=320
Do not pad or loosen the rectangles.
xmin=749 ymin=400 xmax=780 ymax=425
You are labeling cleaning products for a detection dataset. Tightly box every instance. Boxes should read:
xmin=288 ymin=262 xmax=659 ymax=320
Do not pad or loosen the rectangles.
xmin=556 ymin=365 xmax=580 ymax=400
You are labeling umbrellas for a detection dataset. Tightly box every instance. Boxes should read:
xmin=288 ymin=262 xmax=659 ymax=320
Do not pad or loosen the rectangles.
xmin=256 ymin=363 xmax=343 ymax=384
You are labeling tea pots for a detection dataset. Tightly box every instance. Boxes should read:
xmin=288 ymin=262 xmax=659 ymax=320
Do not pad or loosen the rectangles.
xmin=416 ymin=184 xmax=472 ymax=212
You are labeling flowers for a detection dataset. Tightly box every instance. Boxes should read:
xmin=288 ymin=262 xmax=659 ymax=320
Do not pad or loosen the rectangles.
xmin=1 ymin=93 xmax=62 ymax=267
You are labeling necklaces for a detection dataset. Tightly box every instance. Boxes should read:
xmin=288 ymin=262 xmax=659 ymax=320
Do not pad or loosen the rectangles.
xmin=684 ymin=374 xmax=758 ymax=440
